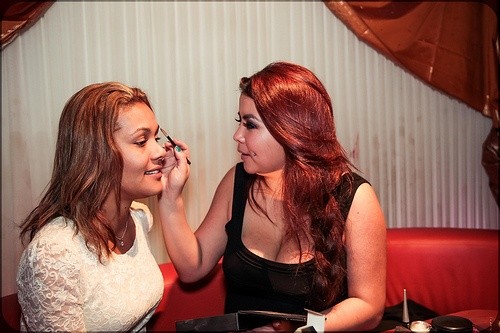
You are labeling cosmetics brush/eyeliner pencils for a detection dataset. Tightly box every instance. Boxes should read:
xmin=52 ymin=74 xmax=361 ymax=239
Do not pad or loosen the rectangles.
xmin=159 ymin=127 xmax=192 ymax=166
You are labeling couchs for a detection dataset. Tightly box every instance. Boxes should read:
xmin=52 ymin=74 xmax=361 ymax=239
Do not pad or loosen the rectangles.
xmin=1 ymin=228 xmax=500 ymax=332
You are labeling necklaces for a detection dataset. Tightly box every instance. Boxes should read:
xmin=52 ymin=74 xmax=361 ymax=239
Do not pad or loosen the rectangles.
xmin=115 ymin=220 xmax=128 ymax=246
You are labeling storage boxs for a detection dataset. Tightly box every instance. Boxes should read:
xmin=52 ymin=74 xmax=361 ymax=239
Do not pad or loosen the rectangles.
xmin=176 ymin=311 xmax=327 ymax=332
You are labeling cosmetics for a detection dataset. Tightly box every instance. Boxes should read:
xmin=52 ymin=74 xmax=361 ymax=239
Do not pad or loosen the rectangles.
xmin=395 ymin=289 xmax=474 ymax=333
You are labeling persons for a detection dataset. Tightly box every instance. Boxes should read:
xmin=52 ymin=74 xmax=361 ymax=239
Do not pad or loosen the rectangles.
xmin=154 ymin=62 xmax=387 ymax=333
xmin=16 ymin=81 xmax=167 ymax=333
